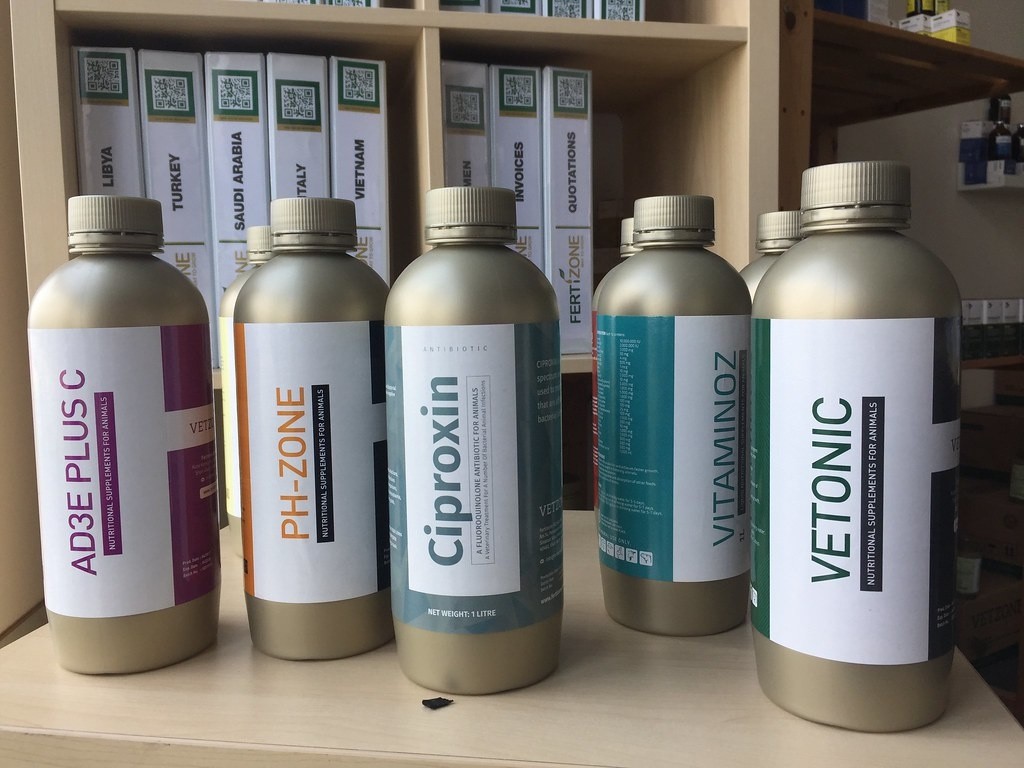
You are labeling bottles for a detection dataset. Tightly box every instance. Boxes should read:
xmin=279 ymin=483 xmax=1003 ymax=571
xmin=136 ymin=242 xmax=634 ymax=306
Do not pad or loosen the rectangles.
xmin=988 ymin=121 xmax=1013 ymax=160
xmin=585 ymin=192 xmax=755 ymax=639
xmin=735 ymin=158 xmax=964 ymax=734
xmin=218 ymin=196 xmax=391 ymax=661
xmin=1012 ymin=123 xmax=1024 ymax=162
xmin=905 ymin=0 xmax=949 ymax=18
xmin=380 ymin=184 xmax=568 ymax=698
xmin=989 ymin=91 xmax=1011 ymax=124
xmin=26 ymin=194 xmax=222 ymax=677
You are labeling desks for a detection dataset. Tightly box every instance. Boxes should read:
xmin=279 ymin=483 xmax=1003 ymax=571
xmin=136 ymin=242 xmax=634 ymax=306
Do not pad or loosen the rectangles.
xmin=0 ymin=513 xmax=1024 ymax=768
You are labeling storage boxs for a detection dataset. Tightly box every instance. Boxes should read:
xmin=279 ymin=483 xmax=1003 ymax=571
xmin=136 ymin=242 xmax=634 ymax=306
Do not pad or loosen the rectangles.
xmin=953 ymin=368 xmax=1024 ymax=727
xmin=814 ymin=0 xmax=970 ymax=46
xmin=837 ymin=91 xmax=1024 ymax=300
xmin=441 ymin=0 xmax=645 ymax=22
xmin=441 ymin=56 xmax=594 ymax=351
xmin=966 ymin=299 xmax=1024 ymax=361
xmin=68 ymin=45 xmax=389 ymax=371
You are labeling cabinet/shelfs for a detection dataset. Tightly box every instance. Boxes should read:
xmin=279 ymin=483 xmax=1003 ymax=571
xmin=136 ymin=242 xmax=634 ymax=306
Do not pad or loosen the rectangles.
xmin=10 ymin=0 xmax=779 ymax=558
xmin=778 ymin=0 xmax=1024 ymax=729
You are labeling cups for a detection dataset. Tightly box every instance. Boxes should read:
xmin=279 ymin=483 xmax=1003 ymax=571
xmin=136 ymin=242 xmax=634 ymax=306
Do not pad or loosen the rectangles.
xmin=957 ymin=557 xmax=982 ymax=595
